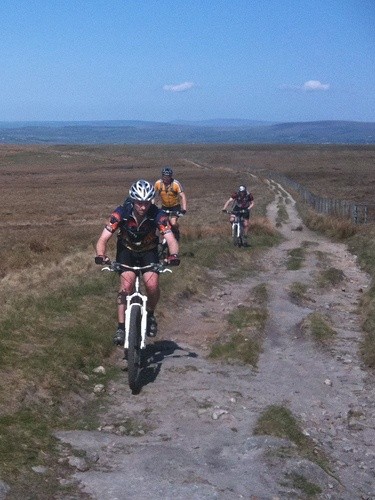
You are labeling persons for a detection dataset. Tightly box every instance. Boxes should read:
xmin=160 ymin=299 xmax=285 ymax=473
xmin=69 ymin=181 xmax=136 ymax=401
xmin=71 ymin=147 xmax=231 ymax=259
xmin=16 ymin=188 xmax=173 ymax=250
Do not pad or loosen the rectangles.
xmin=96 ymin=180 xmax=180 ymax=345
xmin=221 ymin=186 xmax=254 ymax=240
xmin=155 ymin=167 xmax=187 ymax=241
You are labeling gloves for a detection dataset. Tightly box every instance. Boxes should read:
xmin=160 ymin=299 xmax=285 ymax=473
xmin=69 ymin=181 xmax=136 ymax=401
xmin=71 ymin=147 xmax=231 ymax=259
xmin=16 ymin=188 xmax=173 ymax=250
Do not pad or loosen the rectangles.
xmin=95 ymin=255 xmax=105 ymax=264
xmin=168 ymin=255 xmax=180 ymax=266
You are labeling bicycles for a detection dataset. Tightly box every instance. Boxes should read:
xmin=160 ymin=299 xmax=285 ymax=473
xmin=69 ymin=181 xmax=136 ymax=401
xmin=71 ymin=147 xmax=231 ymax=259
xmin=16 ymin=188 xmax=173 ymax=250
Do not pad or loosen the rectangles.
xmin=100 ymin=258 xmax=172 ymax=388
xmin=159 ymin=209 xmax=184 ymax=258
xmin=222 ymin=208 xmax=248 ymax=248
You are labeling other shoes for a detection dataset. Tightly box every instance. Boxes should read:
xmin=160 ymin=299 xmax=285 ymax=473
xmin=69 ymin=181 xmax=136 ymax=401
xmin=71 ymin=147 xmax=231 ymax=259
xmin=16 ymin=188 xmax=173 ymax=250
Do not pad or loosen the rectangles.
xmin=147 ymin=317 xmax=157 ymax=336
xmin=243 ymin=238 xmax=247 ymax=243
xmin=114 ymin=329 xmax=124 ymax=343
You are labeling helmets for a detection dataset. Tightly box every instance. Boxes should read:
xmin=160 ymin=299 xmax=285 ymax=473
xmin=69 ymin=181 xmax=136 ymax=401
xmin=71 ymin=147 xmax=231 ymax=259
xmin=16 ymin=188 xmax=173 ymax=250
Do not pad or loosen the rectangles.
xmin=161 ymin=167 xmax=172 ymax=176
xmin=128 ymin=179 xmax=155 ymax=202
xmin=239 ymin=186 xmax=246 ymax=191
